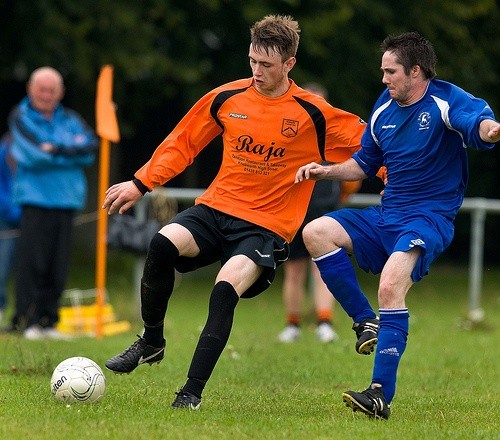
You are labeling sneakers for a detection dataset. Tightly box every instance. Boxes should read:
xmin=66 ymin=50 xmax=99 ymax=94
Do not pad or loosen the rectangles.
xmin=105 ymin=334 xmax=166 ymax=376
xmin=352 ymin=316 xmax=381 ymax=356
xmin=24 ymin=323 xmax=74 ymax=342
xmin=276 ymin=324 xmax=302 ymax=345
xmin=342 ymin=384 xmax=391 ymax=421
xmin=315 ymin=323 xmax=335 ymax=344
xmin=171 ymin=386 xmax=202 ymax=411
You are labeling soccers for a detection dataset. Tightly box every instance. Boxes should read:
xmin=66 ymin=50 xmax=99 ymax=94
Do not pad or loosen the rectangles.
xmin=50 ymin=356 xmax=105 ymax=403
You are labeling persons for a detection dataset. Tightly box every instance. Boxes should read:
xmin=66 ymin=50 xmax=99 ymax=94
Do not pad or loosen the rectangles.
xmin=0 ymin=66 xmax=98 ymax=340
xmin=102 ymin=16 xmax=387 ymax=409
xmin=279 ymin=83 xmax=361 ymax=343
xmin=133 ymin=185 xmax=178 ymax=314
xmin=294 ymin=32 xmax=500 ymax=421
